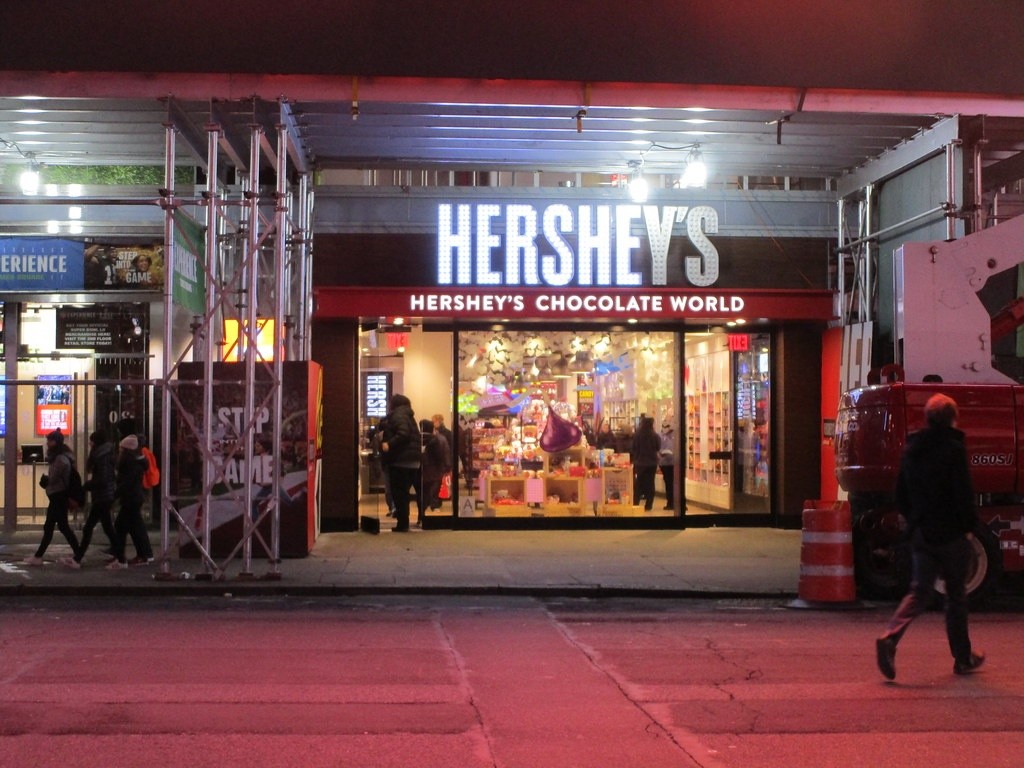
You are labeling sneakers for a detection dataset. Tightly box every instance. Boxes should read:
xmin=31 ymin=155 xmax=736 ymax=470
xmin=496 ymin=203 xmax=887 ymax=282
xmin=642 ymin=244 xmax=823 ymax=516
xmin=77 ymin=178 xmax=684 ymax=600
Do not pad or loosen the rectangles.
xmin=105 ymin=559 xmax=128 ymax=569
xmin=128 ymin=558 xmax=148 ymax=566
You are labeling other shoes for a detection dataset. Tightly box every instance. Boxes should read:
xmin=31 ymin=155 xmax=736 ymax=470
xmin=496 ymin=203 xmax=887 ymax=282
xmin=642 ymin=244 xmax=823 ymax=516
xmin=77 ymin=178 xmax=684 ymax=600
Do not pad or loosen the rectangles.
xmin=954 ymin=652 xmax=985 ymax=673
xmin=148 ymin=551 xmax=154 ymax=561
xmin=23 ymin=556 xmax=43 ymax=565
xmin=391 ymin=527 xmax=409 ymax=532
xmin=877 ymin=638 xmax=897 ymax=679
xmin=99 ymin=549 xmax=115 ymax=558
xmin=386 ymin=511 xmax=396 ymax=517
xmin=60 ymin=558 xmax=80 ymax=569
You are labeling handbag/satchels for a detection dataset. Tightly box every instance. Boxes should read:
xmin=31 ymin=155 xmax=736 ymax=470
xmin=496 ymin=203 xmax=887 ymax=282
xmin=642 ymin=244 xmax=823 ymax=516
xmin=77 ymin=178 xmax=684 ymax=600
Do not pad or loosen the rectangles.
xmin=438 ymin=473 xmax=452 ymax=499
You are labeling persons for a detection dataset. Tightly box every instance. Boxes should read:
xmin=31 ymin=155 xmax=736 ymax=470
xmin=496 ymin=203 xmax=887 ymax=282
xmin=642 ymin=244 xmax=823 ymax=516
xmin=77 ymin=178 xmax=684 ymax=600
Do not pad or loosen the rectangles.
xmin=23 ymin=428 xmax=87 ymax=564
xmin=61 ymin=431 xmax=117 ymax=566
xmin=419 ymin=413 xmax=469 ymax=511
xmin=84 ymin=245 xmax=163 ymax=289
xmin=630 ymin=416 xmax=661 ymax=509
xmin=583 ymin=421 xmax=646 ymax=506
xmin=105 ymin=418 xmax=154 ymax=569
xmin=380 ymin=393 xmax=420 ymax=532
xmin=38 ymin=384 xmax=69 ymax=404
xmin=658 ymin=416 xmax=688 ymax=510
xmin=364 ymin=418 xmax=396 ymax=518
xmin=876 ymin=395 xmax=984 ymax=679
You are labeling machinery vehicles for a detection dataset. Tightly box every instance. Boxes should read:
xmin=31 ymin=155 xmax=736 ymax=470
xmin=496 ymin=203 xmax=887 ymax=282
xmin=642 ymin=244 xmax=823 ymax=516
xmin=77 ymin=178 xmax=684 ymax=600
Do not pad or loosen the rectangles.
xmin=834 ymin=213 xmax=1024 ymax=615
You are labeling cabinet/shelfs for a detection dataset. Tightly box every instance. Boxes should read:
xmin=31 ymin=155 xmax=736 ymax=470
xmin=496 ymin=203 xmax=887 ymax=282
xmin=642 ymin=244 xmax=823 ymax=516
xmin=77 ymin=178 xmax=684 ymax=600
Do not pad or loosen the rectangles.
xmin=605 ymin=389 xmax=730 ymax=497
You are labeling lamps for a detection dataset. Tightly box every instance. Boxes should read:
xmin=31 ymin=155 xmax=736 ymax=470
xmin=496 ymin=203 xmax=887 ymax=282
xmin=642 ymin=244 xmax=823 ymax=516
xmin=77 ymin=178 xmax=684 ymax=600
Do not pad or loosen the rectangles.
xmin=682 ymin=143 xmax=707 ymax=187
xmin=628 ymin=159 xmax=651 ymax=202
xmin=485 ymin=328 xmax=651 ymax=387
xmin=131 ymin=317 xmax=141 ymax=335
xmin=0 ymin=137 xmax=44 ymax=195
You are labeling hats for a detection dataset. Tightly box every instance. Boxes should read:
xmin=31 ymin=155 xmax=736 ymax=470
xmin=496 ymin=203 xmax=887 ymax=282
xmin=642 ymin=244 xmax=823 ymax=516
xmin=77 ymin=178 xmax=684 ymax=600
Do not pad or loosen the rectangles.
xmin=120 ymin=434 xmax=138 ymax=450
xmin=89 ymin=429 xmax=107 ymax=443
xmin=46 ymin=428 xmax=64 ymax=445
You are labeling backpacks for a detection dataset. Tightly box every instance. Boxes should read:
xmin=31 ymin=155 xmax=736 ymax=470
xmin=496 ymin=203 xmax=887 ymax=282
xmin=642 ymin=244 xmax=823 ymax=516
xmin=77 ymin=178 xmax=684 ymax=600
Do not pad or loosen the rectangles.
xmin=58 ymin=455 xmax=83 ymax=501
xmin=141 ymin=447 xmax=160 ymax=489
